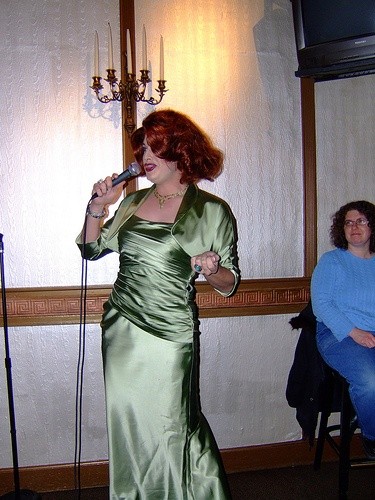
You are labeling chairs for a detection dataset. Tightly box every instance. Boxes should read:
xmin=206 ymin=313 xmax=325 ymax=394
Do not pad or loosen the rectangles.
xmin=316 ymin=370 xmax=375 ymax=500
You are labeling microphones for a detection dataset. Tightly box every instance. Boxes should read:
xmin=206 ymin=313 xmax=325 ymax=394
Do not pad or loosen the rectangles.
xmin=92 ymin=162 xmax=142 ymax=199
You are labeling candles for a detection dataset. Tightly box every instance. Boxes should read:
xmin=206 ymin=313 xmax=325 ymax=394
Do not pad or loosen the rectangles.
xmin=108 ymin=21 xmax=113 ymax=69
xmin=142 ymin=23 xmax=148 ymax=71
xmin=92 ymin=29 xmax=100 ymax=76
xmin=159 ymin=33 xmax=165 ymax=80
xmin=126 ymin=27 xmax=133 ymax=75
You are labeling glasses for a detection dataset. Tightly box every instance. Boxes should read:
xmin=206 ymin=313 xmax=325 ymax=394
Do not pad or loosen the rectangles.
xmin=344 ymin=218 xmax=368 ymax=226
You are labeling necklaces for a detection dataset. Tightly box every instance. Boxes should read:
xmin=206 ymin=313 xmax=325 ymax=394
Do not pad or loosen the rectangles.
xmin=154 ymin=183 xmax=190 ymax=208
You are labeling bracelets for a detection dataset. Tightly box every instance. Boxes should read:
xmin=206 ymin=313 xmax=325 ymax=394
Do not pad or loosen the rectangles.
xmin=86 ymin=203 xmax=107 ymax=218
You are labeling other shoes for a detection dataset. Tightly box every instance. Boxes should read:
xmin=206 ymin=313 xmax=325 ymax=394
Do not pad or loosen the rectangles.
xmin=362 ymin=436 xmax=375 ymax=460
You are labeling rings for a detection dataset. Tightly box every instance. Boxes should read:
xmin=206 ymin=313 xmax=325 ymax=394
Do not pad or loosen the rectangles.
xmin=194 ymin=265 xmax=204 ymax=272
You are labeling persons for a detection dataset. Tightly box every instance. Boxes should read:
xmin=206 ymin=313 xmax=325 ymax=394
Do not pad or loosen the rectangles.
xmin=75 ymin=109 xmax=242 ymax=500
xmin=310 ymin=200 xmax=375 ymax=460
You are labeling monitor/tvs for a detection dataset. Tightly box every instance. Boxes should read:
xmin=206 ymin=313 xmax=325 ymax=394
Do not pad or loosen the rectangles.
xmin=290 ymin=0 xmax=375 ymax=77
